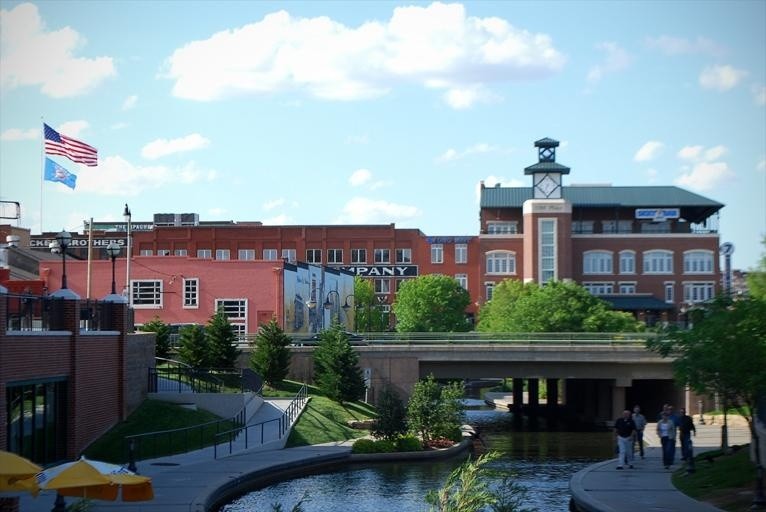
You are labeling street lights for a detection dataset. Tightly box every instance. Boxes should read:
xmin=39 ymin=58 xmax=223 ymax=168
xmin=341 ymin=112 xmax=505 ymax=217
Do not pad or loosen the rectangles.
xmin=342 ymin=294 xmax=371 ymax=332
xmin=55 ymin=229 xmax=72 ymax=288
xmin=123 ymin=203 xmax=131 ymax=308
xmin=105 ymin=243 xmax=121 ymax=294
xmin=323 ymin=290 xmax=341 ymax=330
xmin=305 ymin=287 xmax=325 ymax=332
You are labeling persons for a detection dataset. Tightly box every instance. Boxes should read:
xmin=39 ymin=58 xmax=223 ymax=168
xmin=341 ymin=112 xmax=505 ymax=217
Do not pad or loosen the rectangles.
xmin=657 ymin=402 xmax=673 ymax=423
xmin=662 ymin=406 xmax=679 ymax=464
xmin=676 ymin=408 xmax=696 ymax=461
xmin=629 ymin=405 xmax=647 ymax=459
xmin=19 ymin=287 xmax=32 ymax=332
xmin=41 ymin=285 xmax=49 ymax=330
xmin=615 ymin=410 xmax=636 ymax=469
xmin=656 ymin=412 xmax=677 ymax=470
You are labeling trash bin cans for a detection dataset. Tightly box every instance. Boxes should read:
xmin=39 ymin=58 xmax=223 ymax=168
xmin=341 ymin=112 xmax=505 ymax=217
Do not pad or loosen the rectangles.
xmin=8 ymin=314 xmax=20 ymax=330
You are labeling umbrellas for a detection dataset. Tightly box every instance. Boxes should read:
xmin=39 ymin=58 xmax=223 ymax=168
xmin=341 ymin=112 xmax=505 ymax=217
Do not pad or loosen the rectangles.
xmin=12 ymin=454 xmax=154 ymax=511
xmin=0 ymin=448 xmax=42 ymax=498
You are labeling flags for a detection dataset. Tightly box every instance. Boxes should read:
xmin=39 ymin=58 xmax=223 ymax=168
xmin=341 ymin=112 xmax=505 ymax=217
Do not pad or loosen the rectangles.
xmin=42 ymin=122 xmax=98 ymax=168
xmin=43 ymin=158 xmax=77 ymax=191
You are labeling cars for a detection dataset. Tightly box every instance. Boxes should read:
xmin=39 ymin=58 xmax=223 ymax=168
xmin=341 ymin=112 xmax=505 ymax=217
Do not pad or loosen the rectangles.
xmin=295 ymin=330 xmax=369 ymax=346
